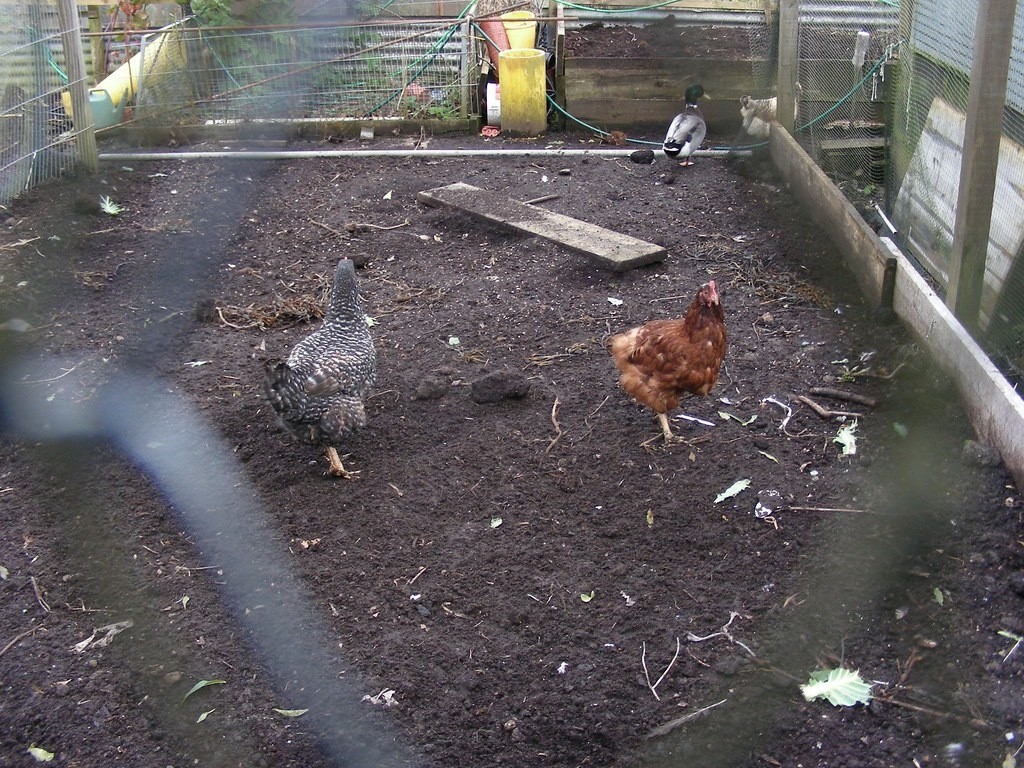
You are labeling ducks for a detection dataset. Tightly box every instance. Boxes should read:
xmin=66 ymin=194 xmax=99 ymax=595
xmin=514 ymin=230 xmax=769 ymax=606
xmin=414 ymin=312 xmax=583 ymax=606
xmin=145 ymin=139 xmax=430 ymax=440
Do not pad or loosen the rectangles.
xmin=662 ymin=84 xmax=712 ymax=166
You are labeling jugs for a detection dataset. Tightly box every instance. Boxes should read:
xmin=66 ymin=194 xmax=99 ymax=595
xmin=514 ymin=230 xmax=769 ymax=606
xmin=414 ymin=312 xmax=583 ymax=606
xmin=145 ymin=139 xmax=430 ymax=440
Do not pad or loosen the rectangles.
xmin=88 ymin=88 xmax=129 ymax=130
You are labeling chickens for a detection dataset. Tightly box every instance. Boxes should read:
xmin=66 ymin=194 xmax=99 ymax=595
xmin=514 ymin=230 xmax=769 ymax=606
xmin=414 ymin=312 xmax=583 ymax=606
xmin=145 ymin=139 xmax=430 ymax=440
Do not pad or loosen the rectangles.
xmin=262 ymin=256 xmax=378 ymax=480
xmin=606 ymin=280 xmax=726 ymax=446
xmin=739 ymin=82 xmax=804 ymax=140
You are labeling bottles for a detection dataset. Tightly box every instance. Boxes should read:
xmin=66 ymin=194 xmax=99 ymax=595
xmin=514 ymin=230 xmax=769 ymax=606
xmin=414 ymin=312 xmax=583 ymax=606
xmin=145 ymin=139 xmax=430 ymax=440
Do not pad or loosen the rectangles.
xmin=852 ymin=32 xmax=870 ymax=66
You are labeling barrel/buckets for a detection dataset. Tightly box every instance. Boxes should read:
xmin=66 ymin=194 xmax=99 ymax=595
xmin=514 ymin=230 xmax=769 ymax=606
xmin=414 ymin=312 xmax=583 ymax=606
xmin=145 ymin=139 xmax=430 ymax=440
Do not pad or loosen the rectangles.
xmin=487 ymin=83 xmax=502 ymax=126
xmin=500 ymin=11 xmax=537 ymax=49
xmin=498 ymin=49 xmax=546 ymax=137
